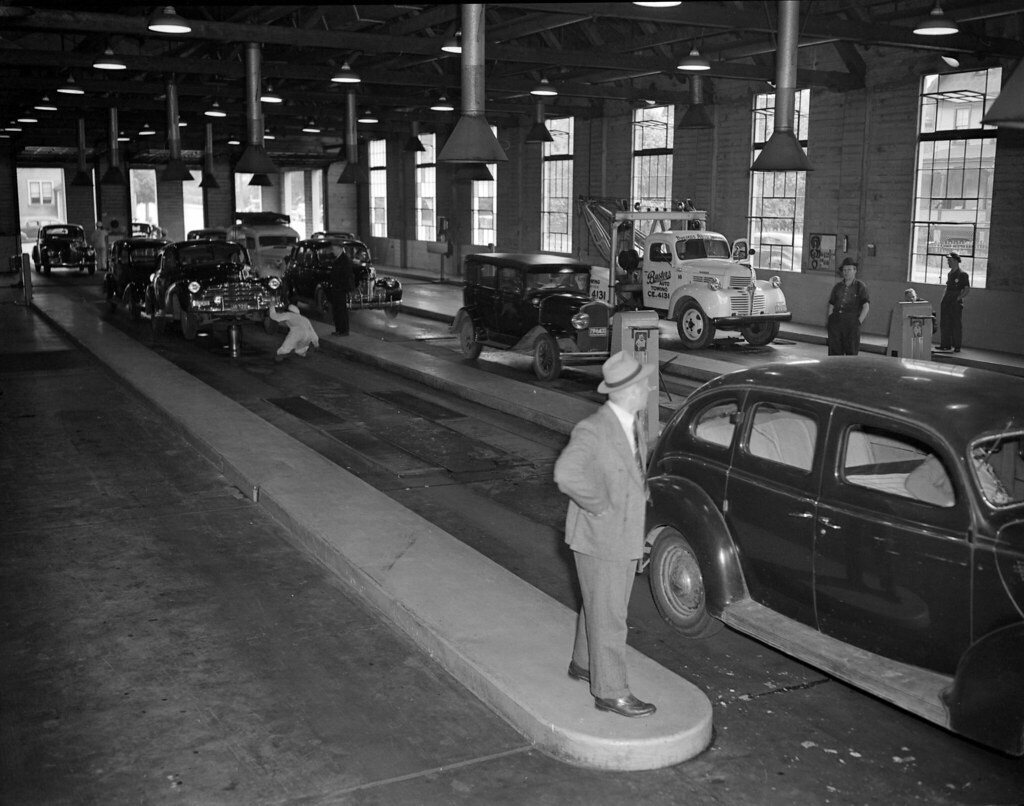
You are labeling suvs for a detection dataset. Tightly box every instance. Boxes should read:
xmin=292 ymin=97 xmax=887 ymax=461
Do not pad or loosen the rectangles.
xmin=100 ymin=233 xmax=175 ymax=323
xmin=32 ymin=222 xmax=99 ymax=275
xmin=456 ymin=249 xmax=618 ymax=383
xmin=230 ymin=211 xmax=301 ymax=273
xmin=151 ymin=228 xmax=284 ymax=339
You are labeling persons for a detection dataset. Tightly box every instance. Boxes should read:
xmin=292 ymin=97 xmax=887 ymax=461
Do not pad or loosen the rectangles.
xmin=906 ymin=443 xmax=1009 ymax=508
xmin=827 ymin=257 xmax=870 ymax=356
xmin=269 ymin=300 xmax=319 ymax=357
xmin=327 ymin=242 xmax=350 ymax=336
xmin=905 ymin=288 xmax=924 ymax=301
xmin=935 ymin=252 xmax=970 ymax=352
xmin=544 ymin=273 xmax=565 ymax=288
xmin=553 ymin=351 xmax=656 ymax=718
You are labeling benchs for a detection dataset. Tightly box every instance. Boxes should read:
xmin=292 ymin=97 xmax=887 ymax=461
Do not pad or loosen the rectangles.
xmin=696 ymin=409 xmax=875 ymax=471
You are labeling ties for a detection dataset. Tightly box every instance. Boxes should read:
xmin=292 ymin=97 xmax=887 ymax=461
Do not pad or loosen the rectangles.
xmin=634 ymin=422 xmax=646 ymax=484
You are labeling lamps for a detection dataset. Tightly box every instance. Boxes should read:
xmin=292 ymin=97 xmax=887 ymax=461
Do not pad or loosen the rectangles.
xmin=441 ymin=28 xmax=462 ymax=53
xmin=0 ymin=128 xmax=10 ymax=138
xmin=5 ymin=120 xmax=22 ymax=131
xmin=330 ymin=62 xmax=361 ymax=83
xmin=530 ymin=75 xmax=558 ymax=96
xmin=204 ymin=100 xmax=226 ymax=116
xmin=117 ymin=131 xmax=130 ymax=141
xmin=358 ymin=110 xmax=378 ymax=123
xmin=93 ymin=46 xmax=126 ymax=69
xmin=302 ymin=120 xmax=320 ymax=132
xmin=179 ymin=115 xmax=187 ymax=127
xmin=430 ymin=96 xmax=454 ymax=111
xmin=17 ymin=109 xmax=37 ymax=123
xmin=148 ymin=5 xmax=191 ymax=33
xmin=263 ymin=129 xmax=275 ymax=139
xmin=260 ymin=78 xmax=282 ymax=103
xmin=34 ymin=94 xmax=57 ymax=111
xmin=912 ymin=0 xmax=959 ymax=35
xmin=677 ymin=37 xmax=711 ymax=71
xmin=57 ymin=75 xmax=84 ymax=94
xmin=138 ymin=123 xmax=155 ymax=135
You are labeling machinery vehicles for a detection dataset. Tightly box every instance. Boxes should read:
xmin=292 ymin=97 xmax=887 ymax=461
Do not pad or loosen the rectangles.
xmin=575 ymin=194 xmax=800 ymax=350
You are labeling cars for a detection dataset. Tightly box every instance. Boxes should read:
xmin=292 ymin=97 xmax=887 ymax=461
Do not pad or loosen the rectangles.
xmin=645 ymin=358 xmax=1024 ymax=770
xmin=287 ymin=230 xmax=409 ymax=317
xmin=190 ymin=228 xmax=228 ymax=243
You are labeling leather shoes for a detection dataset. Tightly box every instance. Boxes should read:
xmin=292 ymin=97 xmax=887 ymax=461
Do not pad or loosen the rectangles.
xmin=568 ymin=660 xmax=592 ymax=682
xmin=592 ymin=684 xmax=658 ymax=716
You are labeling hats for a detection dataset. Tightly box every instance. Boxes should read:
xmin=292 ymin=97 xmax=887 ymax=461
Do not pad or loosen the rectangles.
xmin=945 ymin=251 xmax=961 ymax=262
xmin=839 ymin=257 xmax=859 ymax=271
xmin=597 ymin=349 xmax=654 ymax=395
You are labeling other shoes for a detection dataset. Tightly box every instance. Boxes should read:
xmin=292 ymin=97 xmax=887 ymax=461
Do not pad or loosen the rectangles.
xmin=936 ymin=345 xmax=950 ymax=349
xmin=955 ymin=347 xmax=960 ymax=353
